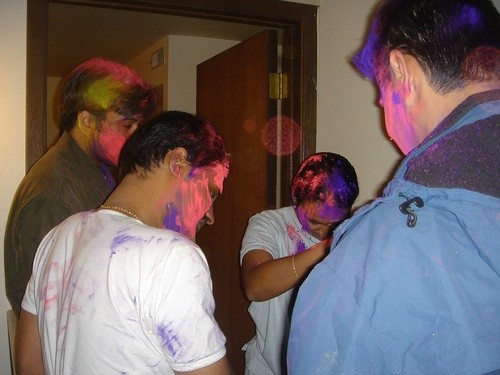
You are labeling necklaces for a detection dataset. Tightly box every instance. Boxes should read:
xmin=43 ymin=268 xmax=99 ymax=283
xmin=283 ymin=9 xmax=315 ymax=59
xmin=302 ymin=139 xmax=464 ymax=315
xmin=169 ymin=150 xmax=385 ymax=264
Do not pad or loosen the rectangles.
xmin=100 ymin=200 xmax=146 ymax=222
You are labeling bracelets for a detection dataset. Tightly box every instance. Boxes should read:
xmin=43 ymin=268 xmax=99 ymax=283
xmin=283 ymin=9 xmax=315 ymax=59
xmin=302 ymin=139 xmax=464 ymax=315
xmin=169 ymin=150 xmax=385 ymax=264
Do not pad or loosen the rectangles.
xmin=290 ymin=254 xmax=305 ymax=285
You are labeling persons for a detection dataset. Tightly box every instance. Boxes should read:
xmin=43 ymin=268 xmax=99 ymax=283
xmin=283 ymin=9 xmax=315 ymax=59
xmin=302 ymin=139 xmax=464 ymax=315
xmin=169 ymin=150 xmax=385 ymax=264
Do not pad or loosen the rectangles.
xmin=281 ymin=0 xmax=500 ymax=374
xmin=235 ymin=151 xmax=361 ymax=374
xmin=4 ymin=54 xmax=160 ymax=322
xmin=15 ymin=110 xmax=240 ymax=375
